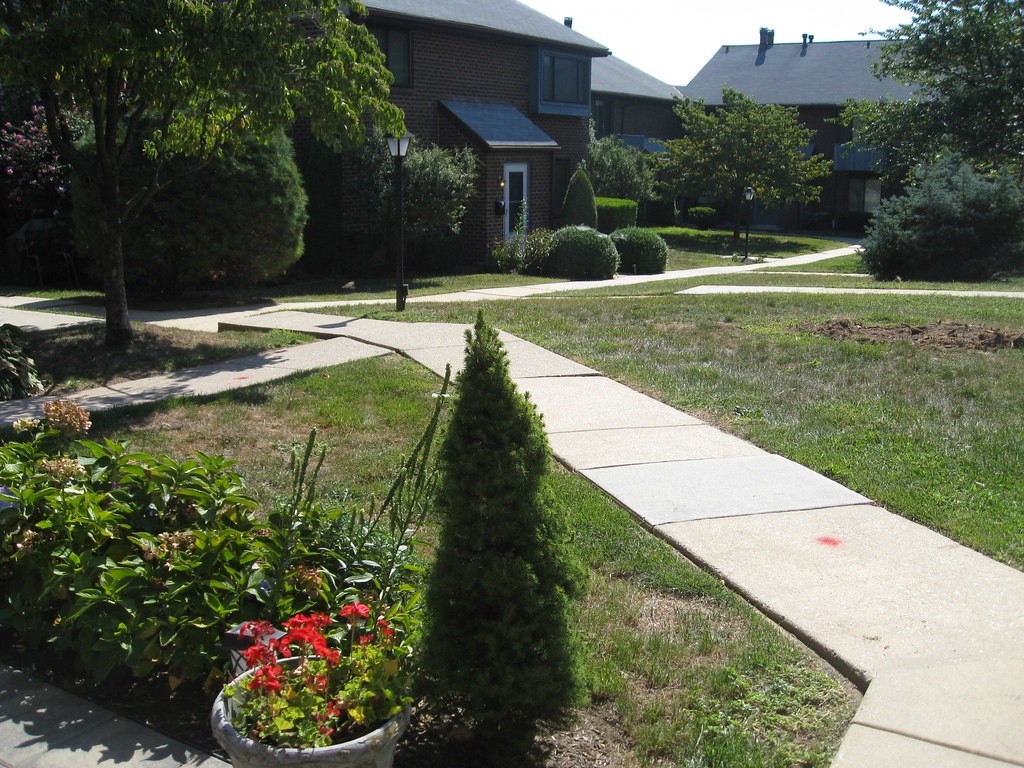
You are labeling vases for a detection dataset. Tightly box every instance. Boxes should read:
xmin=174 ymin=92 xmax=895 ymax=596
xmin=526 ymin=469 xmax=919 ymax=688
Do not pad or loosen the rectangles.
xmin=211 ymin=653 xmax=412 ymax=768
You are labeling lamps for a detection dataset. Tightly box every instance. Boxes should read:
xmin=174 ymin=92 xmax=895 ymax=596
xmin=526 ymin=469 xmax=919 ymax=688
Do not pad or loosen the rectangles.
xmin=498 ymin=176 xmax=505 ymax=191
xmin=225 ymin=622 xmax=288 ymax=676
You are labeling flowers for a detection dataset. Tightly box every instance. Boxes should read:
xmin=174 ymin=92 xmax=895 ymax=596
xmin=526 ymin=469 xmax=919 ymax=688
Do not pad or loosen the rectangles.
xmin=224 ymin=597 xmax=421 ymax=748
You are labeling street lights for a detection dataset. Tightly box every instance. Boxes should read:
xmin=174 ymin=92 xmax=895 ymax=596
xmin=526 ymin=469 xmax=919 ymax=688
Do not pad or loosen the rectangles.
xmin=742 ymin=185 xmax=757 ymax=259
xmin=380 ymin=123 xmax=416 ymax=310
